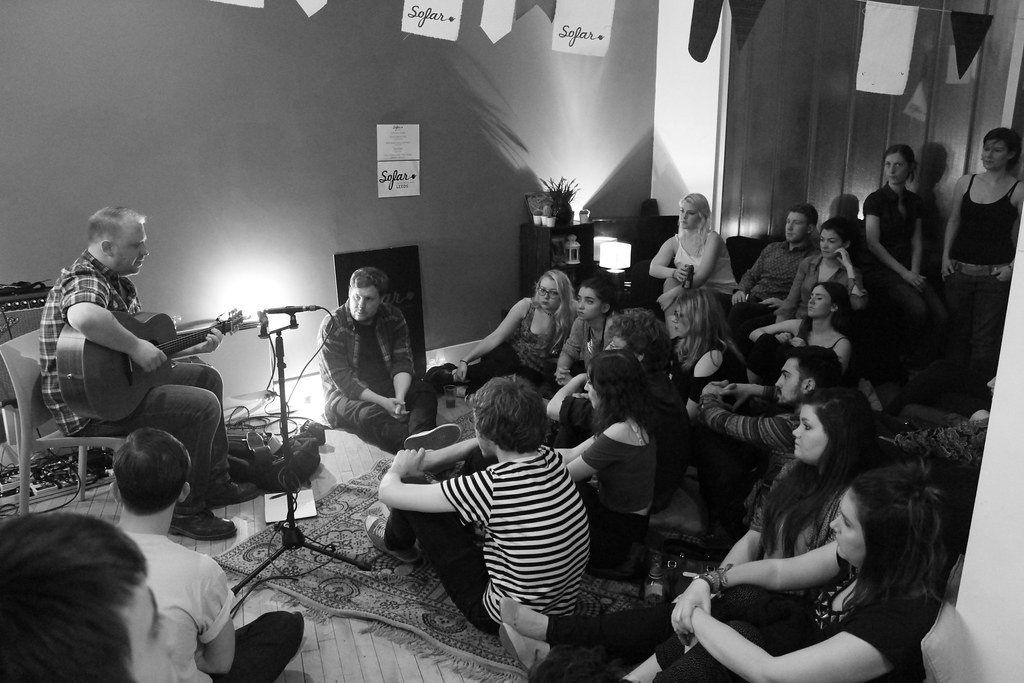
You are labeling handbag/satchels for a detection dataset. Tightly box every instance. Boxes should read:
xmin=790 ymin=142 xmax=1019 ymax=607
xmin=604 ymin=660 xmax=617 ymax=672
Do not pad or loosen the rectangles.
xmin=642 ymin=551 xmax=725 ymax=608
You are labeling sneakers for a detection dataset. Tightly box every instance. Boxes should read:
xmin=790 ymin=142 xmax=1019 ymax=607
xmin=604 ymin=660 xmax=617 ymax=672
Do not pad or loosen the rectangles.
xmin=170 ymin=503 xmax=236 ymax=541
xmin=364 ymin=514 xmax=419 ymax=562
xmin=206 ymin=480 xmax=258 ymax=507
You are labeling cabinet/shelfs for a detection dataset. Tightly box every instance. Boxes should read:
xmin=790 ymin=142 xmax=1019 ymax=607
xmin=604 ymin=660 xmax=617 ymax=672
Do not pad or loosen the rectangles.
xmin=519 ymin=215 xmax=680 ymax=301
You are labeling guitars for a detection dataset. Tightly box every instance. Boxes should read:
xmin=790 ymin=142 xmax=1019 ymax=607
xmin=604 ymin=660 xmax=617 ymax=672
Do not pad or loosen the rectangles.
xmin=55 ymin=309 xmax=244 ymax=423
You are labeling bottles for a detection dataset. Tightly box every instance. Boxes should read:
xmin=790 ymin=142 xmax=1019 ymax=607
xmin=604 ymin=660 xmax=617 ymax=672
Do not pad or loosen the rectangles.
xmin=644 ymin=561 xmax=671 ymax=614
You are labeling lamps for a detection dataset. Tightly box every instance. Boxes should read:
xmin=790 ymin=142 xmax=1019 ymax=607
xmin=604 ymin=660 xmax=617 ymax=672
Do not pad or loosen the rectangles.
xmin=592 ymin=236 xmax=618 ymax=270
xmin=599 ymin=243 xmax=632 ymax=293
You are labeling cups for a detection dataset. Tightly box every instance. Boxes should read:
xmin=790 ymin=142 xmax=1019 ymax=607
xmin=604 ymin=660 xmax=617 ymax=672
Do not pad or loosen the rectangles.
xmin=443 ymin=385 xmax=457 ymax=408
xmin=579 ymin=209 xmax=590 ymax=223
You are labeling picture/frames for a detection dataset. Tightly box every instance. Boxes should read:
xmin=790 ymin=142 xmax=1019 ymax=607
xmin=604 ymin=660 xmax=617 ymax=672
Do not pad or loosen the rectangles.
xmin=524 ymin=191 xmax=554 ymax=223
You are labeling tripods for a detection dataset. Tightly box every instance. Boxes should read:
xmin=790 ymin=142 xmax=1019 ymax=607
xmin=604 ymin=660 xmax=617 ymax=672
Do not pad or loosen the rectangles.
xmin=230 ymin=314 xmax=371 ymax=597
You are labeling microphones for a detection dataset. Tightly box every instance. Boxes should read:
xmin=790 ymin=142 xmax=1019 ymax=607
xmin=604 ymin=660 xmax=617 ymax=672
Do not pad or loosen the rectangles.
xmin=265 ymin=305 xmax=320 ymax=314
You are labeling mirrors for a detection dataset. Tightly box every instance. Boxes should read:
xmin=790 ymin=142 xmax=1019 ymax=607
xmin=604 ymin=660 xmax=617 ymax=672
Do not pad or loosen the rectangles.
xmin=593 ymin=217 xmax=679 ymax=267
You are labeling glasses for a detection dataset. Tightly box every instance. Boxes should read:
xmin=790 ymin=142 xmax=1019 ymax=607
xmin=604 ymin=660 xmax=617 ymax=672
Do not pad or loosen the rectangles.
xmin=674 ymin=310 xmax=687 ymax=321
xmin=538 ymin=286 xmax=561 ymax=299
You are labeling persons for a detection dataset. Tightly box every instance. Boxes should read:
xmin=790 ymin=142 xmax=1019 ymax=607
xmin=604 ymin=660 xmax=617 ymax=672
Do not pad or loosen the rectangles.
xmin=364 ymin=270 xmax=694 ymax=634
xmin=500 ymin=392 xmax=942 ymax=683
xmin=322 ymin=267 xmax=462 ymax=483
xmin=0 ymin=511 xmax=179 ymax=683
xmin=39 ymin=205 xmax=261 ymax=540
xmin=693 ymin=345 xmax=844 ymax=539
xmin=862 ymin=129 xmax=1023 ymax=475
xmin=644 ymin=195 xmax=870 ymax=413
xmin=109 ymin=428 xmax=308 ymax=683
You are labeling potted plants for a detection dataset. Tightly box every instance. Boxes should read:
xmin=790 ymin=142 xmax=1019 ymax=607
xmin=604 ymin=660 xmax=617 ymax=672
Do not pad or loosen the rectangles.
xmin=579 ymin=208 xmax=590 ymax=223
xmin=532 ymin=209 xmax=543 ymax=226
xmin=541 ymin=205 xmax=552 ymax=225
xmin=537 ymin=176 xmax=581 ymax=225
xmin=547 ymin=215 xmax=556 ymax=227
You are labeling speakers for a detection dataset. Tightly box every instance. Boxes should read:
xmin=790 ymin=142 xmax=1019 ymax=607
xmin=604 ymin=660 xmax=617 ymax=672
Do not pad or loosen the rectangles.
xmin=0 ymin=287 xmax=53 ymax=409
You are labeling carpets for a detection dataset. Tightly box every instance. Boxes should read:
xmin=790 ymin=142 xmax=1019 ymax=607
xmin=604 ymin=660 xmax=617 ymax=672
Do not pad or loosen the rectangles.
xmin=212 ymin=409 xmax=639 ymax=683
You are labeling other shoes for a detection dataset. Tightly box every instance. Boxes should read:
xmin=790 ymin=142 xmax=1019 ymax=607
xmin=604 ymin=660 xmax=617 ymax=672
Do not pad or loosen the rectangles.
xmin=403 ymin=423 xmax=462 ymax=452
xmin=500 ymin=622 xmax=538 ymax=672
xmin=499 ymin=597 xmax=520 ymax=629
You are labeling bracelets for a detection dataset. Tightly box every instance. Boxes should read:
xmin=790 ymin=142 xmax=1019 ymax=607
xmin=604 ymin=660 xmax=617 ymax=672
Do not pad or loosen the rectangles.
xmin=672 ymin=270 xmax=676 ymax=278
xmin=460 ymin=360 xmax=468 ymax=365
xmin=699 ymin=564 xmax=734 ymax=593
xmin=1009 ymin=264 xmax=1012 ymax=270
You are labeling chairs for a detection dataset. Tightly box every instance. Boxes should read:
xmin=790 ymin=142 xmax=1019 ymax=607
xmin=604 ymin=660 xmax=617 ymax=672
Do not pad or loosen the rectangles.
xmin=0 ymin=329 xmax=130 ymax=516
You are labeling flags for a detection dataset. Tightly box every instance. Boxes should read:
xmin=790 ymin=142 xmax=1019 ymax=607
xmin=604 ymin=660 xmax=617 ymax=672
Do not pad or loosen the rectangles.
xmin=401 ymin=0 xmax=464 ymax=42
xmin=856 ymin=0 xmax=920 ymax=96
xmin=551 ymin=0 xmax=616 ymax=57
